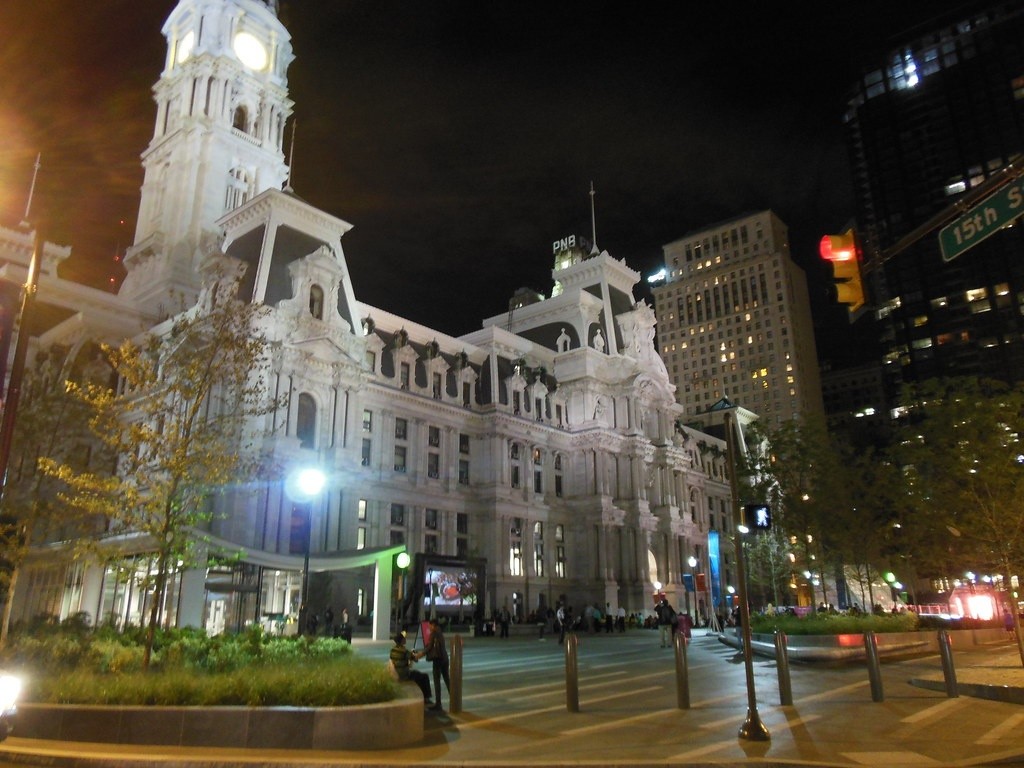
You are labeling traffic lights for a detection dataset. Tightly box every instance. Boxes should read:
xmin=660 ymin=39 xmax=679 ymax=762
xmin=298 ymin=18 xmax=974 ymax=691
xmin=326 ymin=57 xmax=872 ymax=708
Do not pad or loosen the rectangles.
xmin=738 ymin=504 xmax=773 ymax=535
xmin=819 ymin=228 xmax=872 ymax=326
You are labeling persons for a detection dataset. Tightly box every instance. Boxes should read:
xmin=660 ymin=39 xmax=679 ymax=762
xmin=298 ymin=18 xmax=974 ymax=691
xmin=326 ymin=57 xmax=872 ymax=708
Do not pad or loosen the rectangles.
xmin=500 ymin=593 xmax=914 ymax=648
xmin=390 ymin=618 xmax=451 ymax=710
xmin=1002 ymin=609 xmax=1015 ymax=640
xmin=306 ymin=607 xmax=352 ymax=647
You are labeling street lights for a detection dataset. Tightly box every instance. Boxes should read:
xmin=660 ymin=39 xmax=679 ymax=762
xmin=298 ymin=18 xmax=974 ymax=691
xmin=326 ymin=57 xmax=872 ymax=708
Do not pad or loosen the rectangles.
xmin=295 ymin=467 xmax=327 ymax=635
xmin=654 ymin=582 xmax=662 ymax=595
xmin=727 ymin=585 xmax=736 ymax=619
xmin=688 ymin=556 xmax=701 ymax=629
xmin=396 ymin=551 xmax=410 ymax=634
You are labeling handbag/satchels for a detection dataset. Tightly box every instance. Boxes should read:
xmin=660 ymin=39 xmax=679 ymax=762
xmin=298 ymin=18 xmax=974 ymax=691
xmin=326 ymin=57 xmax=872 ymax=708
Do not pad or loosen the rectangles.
xmin=425 ymin=646 xmax=443 ymax=661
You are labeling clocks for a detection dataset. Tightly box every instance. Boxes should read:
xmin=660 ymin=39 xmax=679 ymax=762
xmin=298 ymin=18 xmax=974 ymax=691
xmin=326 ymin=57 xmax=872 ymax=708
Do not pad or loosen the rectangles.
xmin=233 ymin=31 xmax=267 ymax=72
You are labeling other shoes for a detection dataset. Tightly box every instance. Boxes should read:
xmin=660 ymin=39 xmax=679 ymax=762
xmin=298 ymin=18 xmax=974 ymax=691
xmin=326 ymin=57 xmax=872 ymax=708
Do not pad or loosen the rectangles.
xmin=668 ymin=645 xmax=672 ymax=647
xmin=433 ymin=704 xmax=443 ymax=710
xmin=539 ymin=638 xmax=546 ymax=641
xmin=424 ymin=701 xmax=434 ymax=707
xmin=611 ymin=631 xmax=613 ymax=633
xmin=737 ymin=651 xmax=743 ymax=654
xmin=660 ymin=645 xmax=666 ymax=648
xmin=605 ymin=631 xmax=608 ymax=633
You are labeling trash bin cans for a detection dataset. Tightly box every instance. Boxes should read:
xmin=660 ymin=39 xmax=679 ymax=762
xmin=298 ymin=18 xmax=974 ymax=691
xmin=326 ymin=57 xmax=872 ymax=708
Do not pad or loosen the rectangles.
xmin=469 ymin=625 xmax=478 ymax=637
xmin=486 ymin=623 xmax=494 ymax=636
xmin=334 ymin=624 xmax=352 ymax=643
